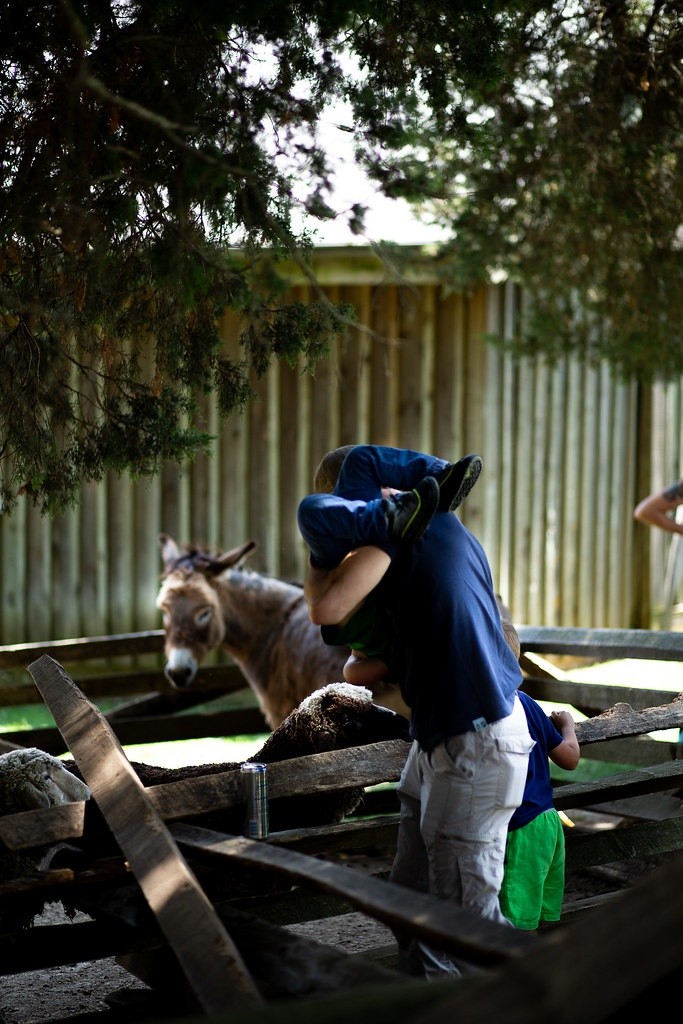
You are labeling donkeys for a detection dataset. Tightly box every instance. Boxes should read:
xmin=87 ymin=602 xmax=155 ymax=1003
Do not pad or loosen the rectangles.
xmin=154 ymin=533 xmax=410 ymax=731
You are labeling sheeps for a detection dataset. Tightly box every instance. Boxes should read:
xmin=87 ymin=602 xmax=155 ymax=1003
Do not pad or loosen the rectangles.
xmin=1 ymin=747 xmax=92 ymax=935
xmin=54 ymin=682 xmax=411 ymax=835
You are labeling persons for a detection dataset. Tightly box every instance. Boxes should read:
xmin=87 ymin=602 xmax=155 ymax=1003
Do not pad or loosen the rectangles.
xmin=633 ymin=478 xmax=683 ymax=535
xmin=313 ymin=447 xmax=533 ymax=984
xmin=497 ymin=619 xmax=580 ymax=939
xmin=298 ymin=444 xmax=485 ymax=695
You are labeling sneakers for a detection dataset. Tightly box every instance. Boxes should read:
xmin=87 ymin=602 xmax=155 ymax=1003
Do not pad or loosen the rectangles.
xmin=442 ymin=455 xmax=484 ymax=513
xmin=390 ymin=476 xmax=439 ymax=544
